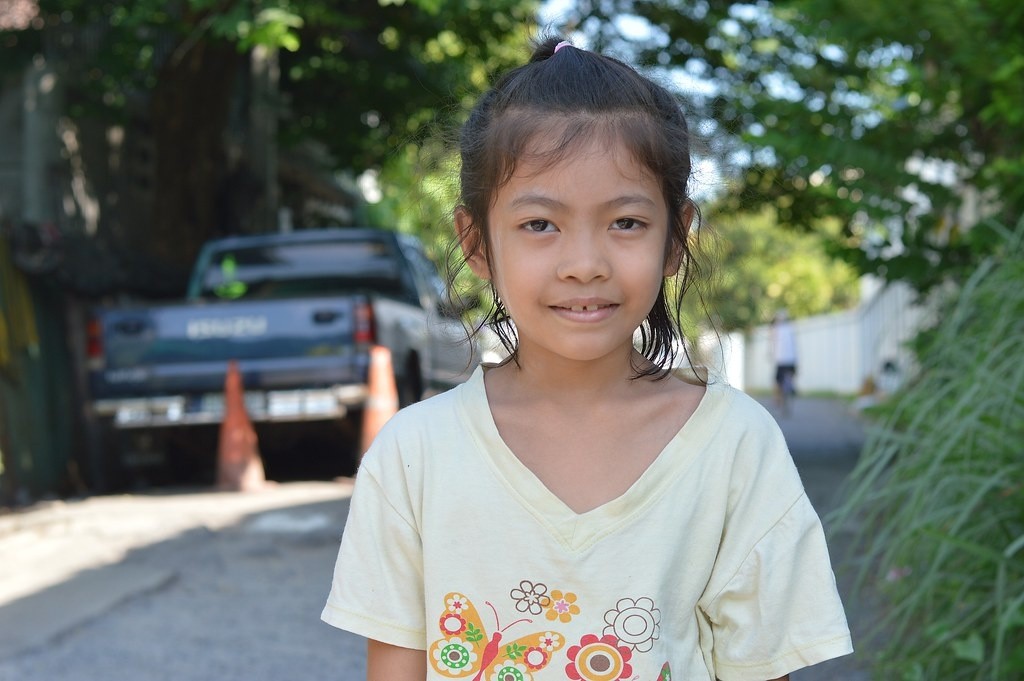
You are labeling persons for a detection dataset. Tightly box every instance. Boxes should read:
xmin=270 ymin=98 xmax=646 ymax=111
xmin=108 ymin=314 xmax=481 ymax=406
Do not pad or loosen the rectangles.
xmin=319 ymin=35 xmax=854 ymax=681
xmin=769 ymin=309 xmax=799 ymax=400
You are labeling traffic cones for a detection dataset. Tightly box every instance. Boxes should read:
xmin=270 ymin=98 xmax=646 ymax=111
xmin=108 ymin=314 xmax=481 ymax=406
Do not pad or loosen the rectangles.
xmin=359 ymin=348 xmax=405 ymax=472
xmin=213 ymin=361 xmax=269 ymax=497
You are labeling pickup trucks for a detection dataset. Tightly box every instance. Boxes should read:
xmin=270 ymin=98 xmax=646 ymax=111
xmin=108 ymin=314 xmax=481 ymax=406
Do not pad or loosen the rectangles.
xmin=87 ymin=228 xmax=487 ymax=501
